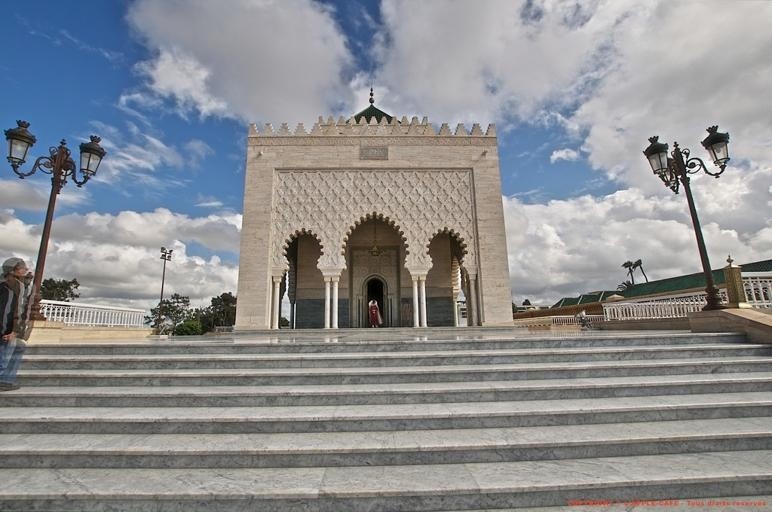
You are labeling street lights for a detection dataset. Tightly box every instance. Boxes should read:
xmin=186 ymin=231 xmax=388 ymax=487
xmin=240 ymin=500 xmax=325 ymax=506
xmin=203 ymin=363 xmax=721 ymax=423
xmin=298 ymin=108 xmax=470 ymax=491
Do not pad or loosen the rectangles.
xmin=4 ymin=120 xmax=107 ymax=345
xmin=152 ymin=247 xmax=173 ymax=333
xmin=643 ymin=124 xmax=730 ymax=312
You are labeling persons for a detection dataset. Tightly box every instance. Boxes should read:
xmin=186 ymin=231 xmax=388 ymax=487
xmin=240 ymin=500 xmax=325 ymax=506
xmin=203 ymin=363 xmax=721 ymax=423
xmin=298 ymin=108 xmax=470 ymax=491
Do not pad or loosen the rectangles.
xmin=368 ymin=296 xmax=384 ymax=328
xmin=0 ymin=257 xmax=33 ymax=391
